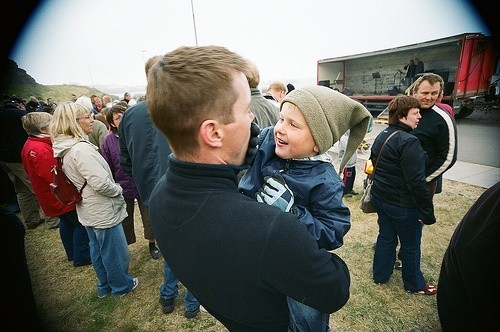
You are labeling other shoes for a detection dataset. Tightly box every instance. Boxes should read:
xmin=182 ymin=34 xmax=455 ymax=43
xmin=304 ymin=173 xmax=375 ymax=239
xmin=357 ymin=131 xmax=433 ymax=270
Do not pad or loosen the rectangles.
xmin=406 ymin=282 xmax=437 ymax=296
xmin=343 ymin=190 xmax=358 ymax=198
xmin=393 ymin=254 xmax=403 ymax=269
xmin=149 ymin=246 xmax=162 ymax=260
xmin=162 ymin=293 xmax=178 ymax=313
xmin=117 ymin=277 xmax=138 ymax=298
xmin=185 ymin=308 xmax=200 ymax=318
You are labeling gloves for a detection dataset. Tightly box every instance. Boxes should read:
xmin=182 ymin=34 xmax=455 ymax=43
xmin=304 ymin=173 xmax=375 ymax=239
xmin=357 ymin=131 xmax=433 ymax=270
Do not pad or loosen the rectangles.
xmin=253 ymin=171 xmax=297 ymax=218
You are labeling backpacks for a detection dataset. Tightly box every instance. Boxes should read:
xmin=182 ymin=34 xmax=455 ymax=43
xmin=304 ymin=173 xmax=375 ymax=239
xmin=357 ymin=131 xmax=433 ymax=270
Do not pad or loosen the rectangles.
xmin=47 ymin=139 xmax=97 ymax=206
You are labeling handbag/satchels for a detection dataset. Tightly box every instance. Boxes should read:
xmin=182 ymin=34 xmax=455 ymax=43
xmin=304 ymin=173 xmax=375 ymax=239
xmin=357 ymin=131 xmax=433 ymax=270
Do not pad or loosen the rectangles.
xmin=359 ymin=176 xmax=375 ymax=215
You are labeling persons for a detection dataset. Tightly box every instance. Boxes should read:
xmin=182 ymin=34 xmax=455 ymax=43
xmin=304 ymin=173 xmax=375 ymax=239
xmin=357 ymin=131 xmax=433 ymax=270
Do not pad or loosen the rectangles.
xmin=396 ymin=73 xmax=458 ymax=270
xmin=370 ymin=95 xmax=437 ymax=296
xmin=20 ymin=93 xmax=137 ymax=114
xmin=243 ymin=59 xmax=280 ymax=130
xmin=147 ymin=45 xmax=350 ymax=332
xmin=50 ymin=102 xmax=139 ymax=298
xmin=403 ymin=59 xmax=415 ymax=89
xmin=241 ymin=85 xmax=370 ymax=332
xmin=437 ymin=180 xmax=500 ymax=332
xmin=262 ymin=81 xmax=288 ymax=109
xmin=20 ymin=112 xmax=92 ymax=267
xmin=118 ymin=54 xmax=200 ymax=319
xmin=414 ymin=58 xmax=424 ymax=82
xmin=102 ymin=105 xmax=161 ymax=260
xmin=74 ymin=96 xmax=109 ymax=153
xmin=338 ymin=129 xmax=359 ymax=197
xmin=434 ymin=88 xmax=454 ymax=118
xmin=0 ymin=96 xmax=61 ymax=230
xmin=0 ymin=211 xmax=48 ymax=332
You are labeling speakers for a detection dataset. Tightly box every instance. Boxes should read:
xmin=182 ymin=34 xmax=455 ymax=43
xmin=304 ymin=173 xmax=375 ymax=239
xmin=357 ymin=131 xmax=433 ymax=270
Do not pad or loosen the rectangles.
xmin=318 ymin=80 xmax=330 ymax=88
xmin=437 ymin=71 xmax=455 ymax=96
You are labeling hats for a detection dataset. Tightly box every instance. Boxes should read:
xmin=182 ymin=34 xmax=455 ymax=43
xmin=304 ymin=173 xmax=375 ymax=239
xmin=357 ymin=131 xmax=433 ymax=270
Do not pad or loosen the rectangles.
xmin=281 ymin=86 xmax=372 ymax=175
